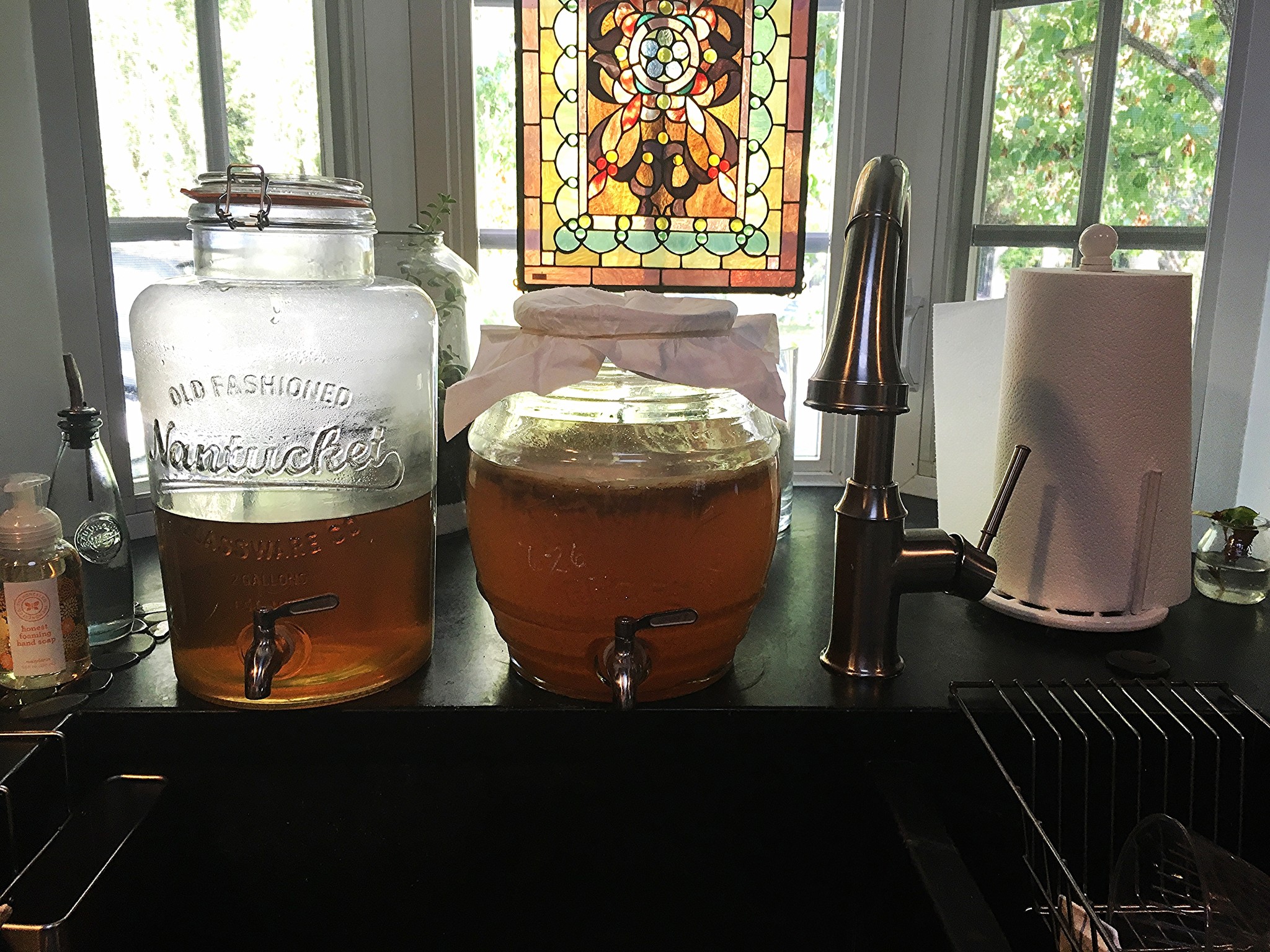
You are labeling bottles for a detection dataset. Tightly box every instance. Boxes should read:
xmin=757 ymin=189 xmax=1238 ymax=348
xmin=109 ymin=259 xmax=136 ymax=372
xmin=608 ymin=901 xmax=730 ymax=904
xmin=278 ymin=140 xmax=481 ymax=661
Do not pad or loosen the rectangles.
xmin=44 ymin=354 xmax=137 ymax=653
xmin=0 ymin=473 xmax=90 ymax=696
xmin=123 ymin=157 xmax=440 ymax=718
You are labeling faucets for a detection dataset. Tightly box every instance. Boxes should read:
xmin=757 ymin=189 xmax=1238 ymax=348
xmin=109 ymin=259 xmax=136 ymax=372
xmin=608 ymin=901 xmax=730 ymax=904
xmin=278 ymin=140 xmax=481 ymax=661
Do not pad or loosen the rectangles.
xmin=242 ymin=594 xmax=340 ymax=700
xmin=802 ymin=156 xmax=1033 ymax=685
xmin=598 ymin=606 xmax=702 ymax=711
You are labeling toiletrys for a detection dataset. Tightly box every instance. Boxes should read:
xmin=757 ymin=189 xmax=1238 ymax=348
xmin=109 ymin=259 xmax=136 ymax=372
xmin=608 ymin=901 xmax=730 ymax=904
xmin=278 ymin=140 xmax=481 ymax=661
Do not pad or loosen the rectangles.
xmin=0 ymin=472 xmax=92 ymax=692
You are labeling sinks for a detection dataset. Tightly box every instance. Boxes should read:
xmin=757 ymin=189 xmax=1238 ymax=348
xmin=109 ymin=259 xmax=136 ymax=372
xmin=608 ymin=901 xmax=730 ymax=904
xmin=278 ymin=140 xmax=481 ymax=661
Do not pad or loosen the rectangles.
xmin=2 ymin=691 xmax=1270 ymax=951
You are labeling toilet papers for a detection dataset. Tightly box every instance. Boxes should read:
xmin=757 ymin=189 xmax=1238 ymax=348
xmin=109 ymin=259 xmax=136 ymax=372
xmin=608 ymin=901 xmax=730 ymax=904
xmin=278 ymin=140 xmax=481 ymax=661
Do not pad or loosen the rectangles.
xmin=930 ymin=264 xmax=1197 ymax=611
